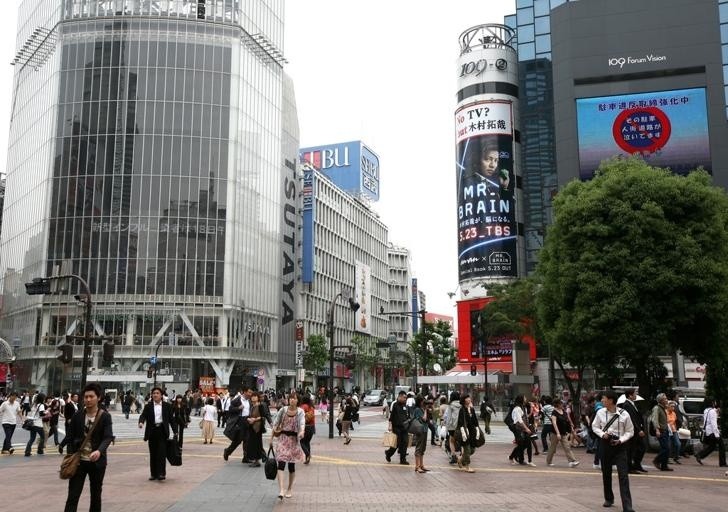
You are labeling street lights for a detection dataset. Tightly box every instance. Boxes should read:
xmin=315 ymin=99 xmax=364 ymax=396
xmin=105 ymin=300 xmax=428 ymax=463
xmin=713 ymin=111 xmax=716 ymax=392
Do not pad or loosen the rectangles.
xmin=472 ymin=313 xmax=495 ymax=413
xmin=326 ymin=291 xmax=360 ymax=444
xmin=378 ymin=305 xmax=429 ymax=397
xmin=153 ymin=341 xmax=166 ymax=388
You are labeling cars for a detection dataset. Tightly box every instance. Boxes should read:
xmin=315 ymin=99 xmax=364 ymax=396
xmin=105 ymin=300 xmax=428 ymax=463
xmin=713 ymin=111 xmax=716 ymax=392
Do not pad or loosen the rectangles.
xmin=363 ymin=389 xmax=388 ymax=406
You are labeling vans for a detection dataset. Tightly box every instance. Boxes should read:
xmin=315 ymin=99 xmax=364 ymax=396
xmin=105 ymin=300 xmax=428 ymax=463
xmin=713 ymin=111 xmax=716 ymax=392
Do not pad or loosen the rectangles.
xmin=615 ymin=386 xmax=706 ymax=450
xmin=385 ymin=384 xmax=414 ymax=408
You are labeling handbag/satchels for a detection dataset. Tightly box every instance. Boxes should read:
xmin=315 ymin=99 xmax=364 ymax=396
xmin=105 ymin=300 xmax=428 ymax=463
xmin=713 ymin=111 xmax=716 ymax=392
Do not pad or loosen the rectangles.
xmin=167 ymin=438 xmax=182 ymax=467
xmin=700 ymin=429 xmax=706 ymax=444
xmin=381 ymin=431 xmax=399 ymax=449
xmin=264 ymin=446 xmax=278 ymax=480
xmin=223 ymin=416 xmax=245 ymax=442
xmin=455 ymin=425 xmax=485 ymax=446
xmin=22 ymin=419 xmax=34 ymax=431
xmin=677 ymin=428 xmax=692 ymax=440
xmin=403 ymin=419 xmax=424 ymax=435
xmin=59 ymin=451 xmax=82 ymax=480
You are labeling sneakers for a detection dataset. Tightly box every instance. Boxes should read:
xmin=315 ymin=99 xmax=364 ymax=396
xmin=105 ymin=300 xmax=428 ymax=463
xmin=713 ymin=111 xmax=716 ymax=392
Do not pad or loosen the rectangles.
xmin=592 ymin=452 xmax=690 ymax=475
xmin=485 ymin=430 xmax=491 ymax=434
xmin=2 ymin=447 xmax=15 ymax=455
xmin=507 ymin=450 xmax=549 ymax=467
xmin=546 ymin=462 xmax=556 ymax=467
xmin=304 ymin=454 xmax=311 ymax=465
xmin=569 ymin=461 xmax=580 ymax=468
xmin=55 ymin=442 xmax=63 ymax=454
xmin=204 ymin=441 xmax=214 ymax=444
xmin=343 ymin=437 xmax=352 ymax=445
xmin=24 ymin=449 xmax=45 ymax=456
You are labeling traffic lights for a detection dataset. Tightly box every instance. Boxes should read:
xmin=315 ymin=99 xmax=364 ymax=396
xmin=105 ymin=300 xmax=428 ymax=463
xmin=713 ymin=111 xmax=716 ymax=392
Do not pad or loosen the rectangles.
xmin=25 ymin=281 xmax=52 ymax=295
xmin=148 ymin=370 xmax=152 ymax=378
xmin=102 ymin=343 xmax=116 ymax=366
xmin=471 ymin=364 xmax=476 ymax=375
xmin=56 ymin=345 xmax=72 ymax=364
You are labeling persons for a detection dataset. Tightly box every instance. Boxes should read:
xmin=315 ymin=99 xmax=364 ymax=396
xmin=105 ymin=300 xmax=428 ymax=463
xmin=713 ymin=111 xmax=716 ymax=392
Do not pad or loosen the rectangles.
xmin=1 ymin=391 xmax=82 ymax=456
xmin=137 ymin=387 xmax=179 ymax=481
xmin=380 ymin=383 xmax=497 ymax=472
xmin=215 ymin=385 xmax=291 ymax=467
xmin=592 ymin=391 xmax=636 ymax=512
xmin=622 ymin=389 xmax=727 ymax=472
xmin=508 ymin=391 xmax=609 ymax=469
xmin=162 ymin=388 xmax=218 ymax=444
xmin=102 ymin=389 xmax=150 ymax=420
xmin=268 ymin=390 xmax=305 ymax=501
xmin=294 ymin=384 xmax=362 ymax=464
xmin=64 ymin=383 xmax=114 ymax=512
xmin=462 ymin=137 xmax=513 ymax=201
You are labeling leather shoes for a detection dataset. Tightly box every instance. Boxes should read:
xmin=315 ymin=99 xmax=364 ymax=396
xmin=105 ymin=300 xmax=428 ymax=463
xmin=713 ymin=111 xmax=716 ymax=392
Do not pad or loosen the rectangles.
xmin=431 ymin=441 xmax=477 ymax=473
xmin=242 ymin=454 xmax=267 ymax=468
xmin=149 ymin=474 xmax=166 ymax=481
xmin=400 ymin=461 xmax=410 ymax=465
xmin=278 ymin=492 xmax=284 ymax=500
xmin=285 ymin=491 xmax=292 ymax=498
xmin=385 ymin=450 xmax=392 ymax=462
xmin=694 ymin=453 xmax=705 ymax=466
xmin=719 ymin=462 xmax=727 ymax=467
xmin=415 ymin=465 xmax=430 ymax=473
xmin=223 ymin=448 xmax=230 ymax=461
xmin=603 ymin=499 xmax=615 ymax=507
